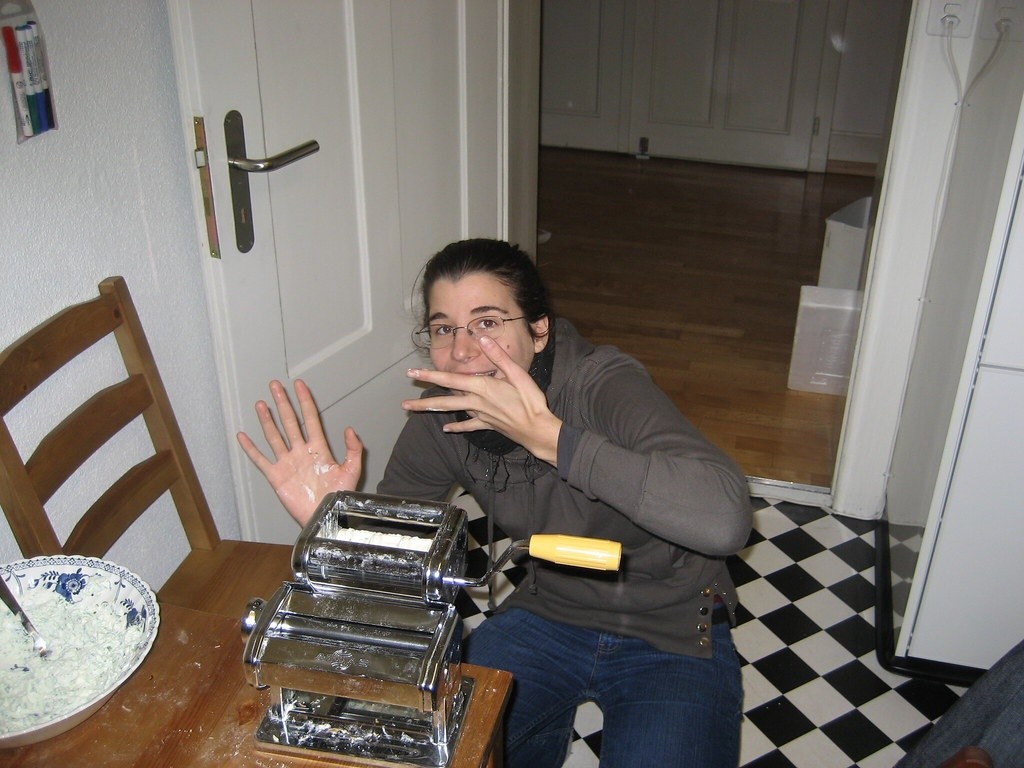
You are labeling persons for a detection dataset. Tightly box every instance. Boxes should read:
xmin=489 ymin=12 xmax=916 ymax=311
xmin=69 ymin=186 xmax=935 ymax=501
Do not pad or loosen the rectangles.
xmin=232 ymin=235 xmax=756 ymax=768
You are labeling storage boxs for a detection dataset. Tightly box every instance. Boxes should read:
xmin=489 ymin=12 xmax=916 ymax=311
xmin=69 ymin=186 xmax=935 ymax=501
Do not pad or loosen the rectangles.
xmin=539 ymin=0 xmax=830 ymax=168
xmin=816 ymin=193 xmax=872 ymax=290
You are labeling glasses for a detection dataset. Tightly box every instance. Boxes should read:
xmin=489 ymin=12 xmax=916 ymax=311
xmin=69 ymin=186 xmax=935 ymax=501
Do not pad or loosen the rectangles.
xmin=411 ymin=316 xmax=526 ymax=349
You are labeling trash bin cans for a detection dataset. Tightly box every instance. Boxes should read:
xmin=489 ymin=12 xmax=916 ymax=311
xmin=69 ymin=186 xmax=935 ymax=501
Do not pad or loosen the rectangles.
xmin=819 ymin=198 xmax=875 ymax=292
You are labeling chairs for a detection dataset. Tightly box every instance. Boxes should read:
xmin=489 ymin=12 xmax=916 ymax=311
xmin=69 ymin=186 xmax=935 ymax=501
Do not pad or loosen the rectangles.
xmin=0 ymin=275 xmax=298 ymax=620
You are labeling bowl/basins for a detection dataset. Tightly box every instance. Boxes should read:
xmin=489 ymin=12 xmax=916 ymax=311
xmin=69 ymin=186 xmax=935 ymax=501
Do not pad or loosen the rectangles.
xmin=0 ymin=555 xmax=160 ymax=748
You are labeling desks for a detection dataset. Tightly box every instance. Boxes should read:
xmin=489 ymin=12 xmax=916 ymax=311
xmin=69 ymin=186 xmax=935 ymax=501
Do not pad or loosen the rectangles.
xmin=0 ymin=603 xmax=514 ymax=768
